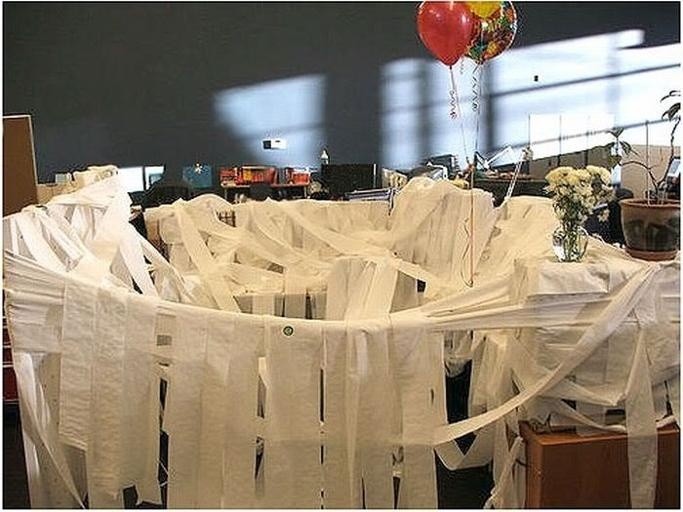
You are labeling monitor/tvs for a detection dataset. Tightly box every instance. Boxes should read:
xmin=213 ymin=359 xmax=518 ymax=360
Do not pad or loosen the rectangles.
xmin=117 ymin=164 xmax=215 ymax=193
xmin=320 ymin=163 xmax=376 ymax=195
xmin=664 ymin=156 xmax=680 ymax=183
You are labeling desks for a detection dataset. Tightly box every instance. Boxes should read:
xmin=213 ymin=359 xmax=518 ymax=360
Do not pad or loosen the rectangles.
xmin=450 ymin=177 xmax=552 ymax=206
xmin=220 ymin=180 xmax=310 ymax=206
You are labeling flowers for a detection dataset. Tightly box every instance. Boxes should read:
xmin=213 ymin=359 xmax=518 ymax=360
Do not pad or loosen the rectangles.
xmin=542 ymin=165 xmax=616 ymax=261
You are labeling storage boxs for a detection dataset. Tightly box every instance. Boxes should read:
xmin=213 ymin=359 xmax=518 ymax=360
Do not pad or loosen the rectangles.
xmin=506 ymin=419 xmax=680 ymax=509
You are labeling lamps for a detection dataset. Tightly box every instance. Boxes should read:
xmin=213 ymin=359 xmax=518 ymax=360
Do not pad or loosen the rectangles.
xmin=321 ymin=149 xmax=329 ymax=164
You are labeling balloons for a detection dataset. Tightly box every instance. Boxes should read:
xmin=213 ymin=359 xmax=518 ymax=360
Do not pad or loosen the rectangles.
xmin=466 ymin=1 xmax=503 ymax=23
xmin=461 ymin=1 xmax=516 ymax=66
xmin=416 ymin=1 xmax=474 ymax=70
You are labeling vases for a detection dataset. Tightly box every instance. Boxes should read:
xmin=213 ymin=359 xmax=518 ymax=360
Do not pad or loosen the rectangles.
xmin=550 ymin=222 xmax=588 ymax=262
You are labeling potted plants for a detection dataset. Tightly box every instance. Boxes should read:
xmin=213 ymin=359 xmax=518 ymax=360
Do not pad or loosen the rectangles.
xmin=602 ymin=90 xmax=680 ymax=260
